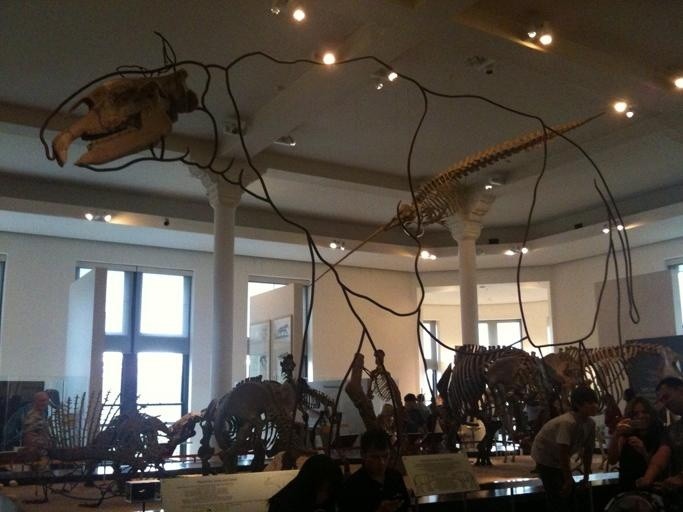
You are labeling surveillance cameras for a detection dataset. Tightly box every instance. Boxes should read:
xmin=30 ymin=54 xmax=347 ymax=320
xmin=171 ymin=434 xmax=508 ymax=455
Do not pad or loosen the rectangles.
xmin=163 ymin=220 xmax=169 ymax=225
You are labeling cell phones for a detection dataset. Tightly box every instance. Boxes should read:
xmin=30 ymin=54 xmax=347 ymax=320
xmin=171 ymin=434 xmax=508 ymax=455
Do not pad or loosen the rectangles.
xmin=393 ymin=496 xmax=405 ymax=503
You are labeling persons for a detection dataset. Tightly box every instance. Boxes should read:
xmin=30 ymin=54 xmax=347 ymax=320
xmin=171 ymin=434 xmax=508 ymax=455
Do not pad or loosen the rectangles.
xmin=23 ymin=390 xmax=53 ymax=468
xmin=530 ymin=388 xmax=598 ymax=512
xmin=267 ymin=453 xmax=344 ymax=511
xmin=602 ymin=393 xmax=625 ymax=465
xmin=624 ymin=388 xmax=635 ymax=417
xmin=378 ymin=394 xmax=470 ymax=511
xmin=608 ymin=397 xmax=672 ymax=511
xmin=654 ymin=400 xmax=671 ymax=427
xmin=655 ymin=377 xmax=682 ymax=511
xmin=344 ymin=429 xmax=412 ymax=512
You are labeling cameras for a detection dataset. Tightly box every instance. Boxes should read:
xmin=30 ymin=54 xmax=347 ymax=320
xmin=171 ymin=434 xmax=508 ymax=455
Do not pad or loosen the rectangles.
xmin=627 ymin=420 xmax=641 ymax=428
